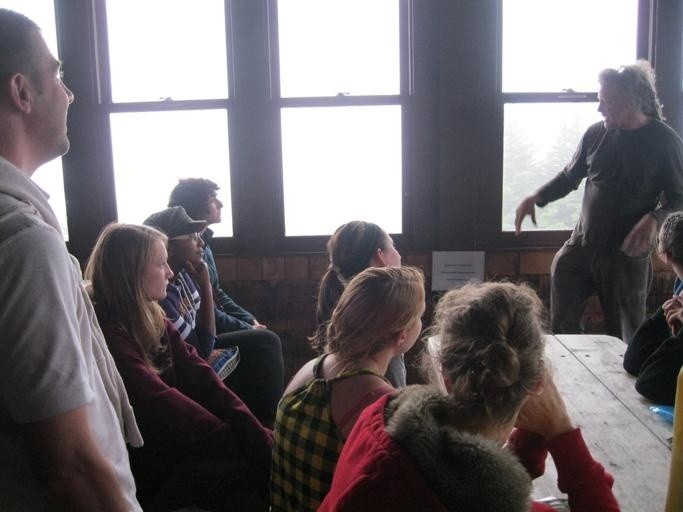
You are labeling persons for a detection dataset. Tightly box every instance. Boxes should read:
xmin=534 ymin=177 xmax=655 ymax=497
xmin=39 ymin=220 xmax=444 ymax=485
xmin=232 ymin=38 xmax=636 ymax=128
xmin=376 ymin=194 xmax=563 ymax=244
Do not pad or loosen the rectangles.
xmin=623 ymin=212 xmax=683 ymax=407
xmin=514 ymin=62 xmax=682 ymax=350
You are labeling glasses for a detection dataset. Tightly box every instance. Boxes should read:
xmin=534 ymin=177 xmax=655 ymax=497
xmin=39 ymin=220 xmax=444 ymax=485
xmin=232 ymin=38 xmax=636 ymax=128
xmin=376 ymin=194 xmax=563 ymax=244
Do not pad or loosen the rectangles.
xmin=171 ymin=232 xmax=201 ymax=240
xmin=617 ymin=66 xmax=632 ymax=77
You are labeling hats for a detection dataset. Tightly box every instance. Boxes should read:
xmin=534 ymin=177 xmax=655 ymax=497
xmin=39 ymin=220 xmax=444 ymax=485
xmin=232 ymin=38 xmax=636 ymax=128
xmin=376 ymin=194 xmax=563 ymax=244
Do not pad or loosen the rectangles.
xmin=143 ymin=206 xmax=207 ymax=237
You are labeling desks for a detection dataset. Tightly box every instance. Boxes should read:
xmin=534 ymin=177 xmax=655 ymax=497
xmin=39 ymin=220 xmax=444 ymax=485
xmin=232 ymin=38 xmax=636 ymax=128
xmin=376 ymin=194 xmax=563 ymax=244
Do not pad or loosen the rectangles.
xmin=424 ymin=334 xmax=681 ymax=510
